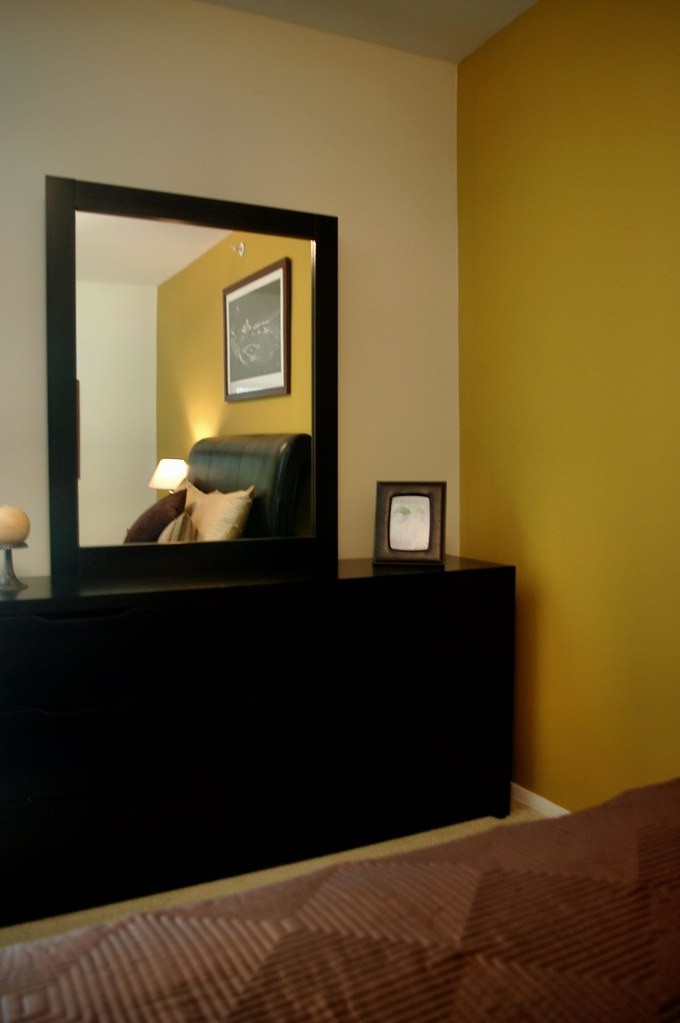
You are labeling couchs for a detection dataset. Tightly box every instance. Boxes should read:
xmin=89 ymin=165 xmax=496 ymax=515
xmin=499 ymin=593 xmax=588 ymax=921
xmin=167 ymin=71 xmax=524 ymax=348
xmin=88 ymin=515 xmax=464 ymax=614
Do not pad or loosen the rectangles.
xmin=123 ymin=432 xmax=312 ymax=546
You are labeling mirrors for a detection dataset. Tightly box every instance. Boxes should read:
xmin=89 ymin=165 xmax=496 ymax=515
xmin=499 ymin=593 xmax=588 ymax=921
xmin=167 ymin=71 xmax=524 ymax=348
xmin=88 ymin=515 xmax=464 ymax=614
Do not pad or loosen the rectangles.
xmin=44 ymin=174 xmax=338 ymax=577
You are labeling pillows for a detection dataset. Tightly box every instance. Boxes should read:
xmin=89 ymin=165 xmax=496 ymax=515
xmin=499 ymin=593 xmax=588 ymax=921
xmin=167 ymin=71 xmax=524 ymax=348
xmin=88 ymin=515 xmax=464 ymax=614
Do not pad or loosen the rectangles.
xmin=157 ymin=513 xmax=196 ymax=544
xmin=185 ymin=479 xmax=255 ymax=544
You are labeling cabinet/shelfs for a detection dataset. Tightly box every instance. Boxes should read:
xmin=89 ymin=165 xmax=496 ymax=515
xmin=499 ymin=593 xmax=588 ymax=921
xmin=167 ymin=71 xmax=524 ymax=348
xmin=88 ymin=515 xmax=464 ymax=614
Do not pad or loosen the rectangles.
xmin=0 ymin=556 xmax=516 ymax=929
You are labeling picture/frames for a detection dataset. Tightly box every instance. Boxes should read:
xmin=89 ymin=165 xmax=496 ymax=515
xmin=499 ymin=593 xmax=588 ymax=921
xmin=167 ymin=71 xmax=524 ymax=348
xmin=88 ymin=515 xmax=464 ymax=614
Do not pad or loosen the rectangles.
xmin=221 ymin=258 xmax=291 ymax=402
xmin=373 ymin=481 xmax=447 ymax=566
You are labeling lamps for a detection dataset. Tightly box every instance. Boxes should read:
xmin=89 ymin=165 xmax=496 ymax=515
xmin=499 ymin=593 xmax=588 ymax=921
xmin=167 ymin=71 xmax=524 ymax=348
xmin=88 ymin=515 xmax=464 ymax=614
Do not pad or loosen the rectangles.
xmin=147 ymin=459 xmax=189 ymax=494
xmin=0 ymin=503 xmax=32 ymax=592
